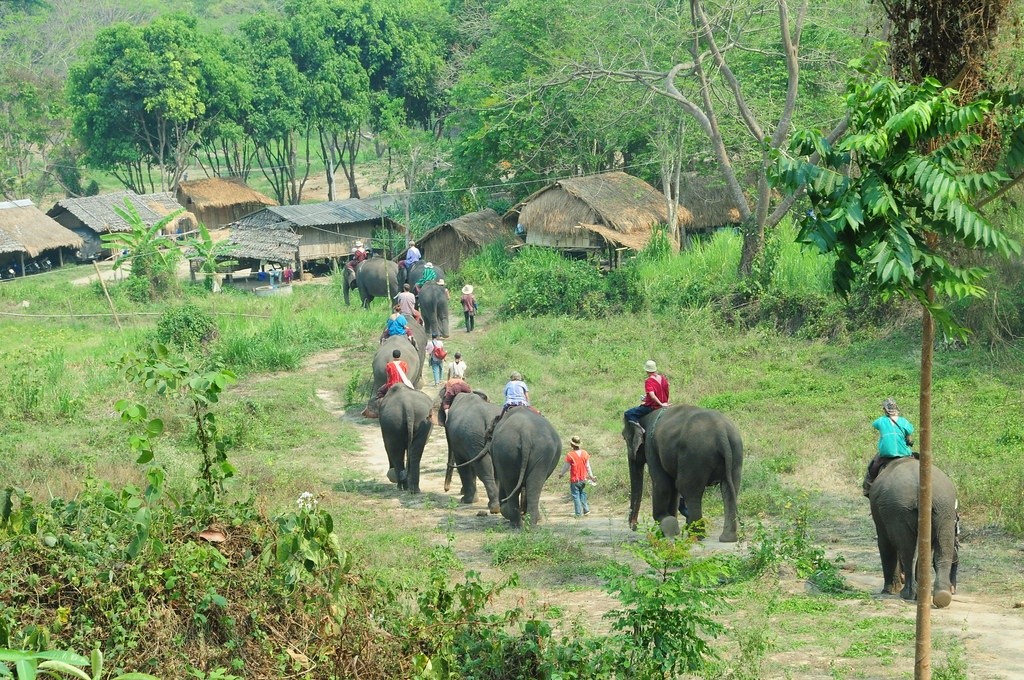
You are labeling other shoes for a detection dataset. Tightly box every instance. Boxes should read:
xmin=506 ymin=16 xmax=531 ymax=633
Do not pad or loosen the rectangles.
xmin=583 ymin=511 xmax=590 ymax=515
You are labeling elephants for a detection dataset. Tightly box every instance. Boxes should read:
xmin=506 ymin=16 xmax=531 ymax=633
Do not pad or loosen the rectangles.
xmin=418 ymin=280 xmax=449 ymax=338
xmin=380 ymin=315 xmax=427 ymax=377
xmin=622 ymin=404 xmax=743 ymax=542
xmin=361 ymin=383 xmax=434 ymax=494
xmin=396 ymin=260 xmax=444 ymax=296
xmin=862 ymin=452 xmax=959 ymax=608
xmin=426 ymin=387 xmax=501 ymax=514
xmin=372 ymin=335 xmax=420 ymax=387
xmin=342 ymin=258 xmax=399 ymax=308
xmin=447 ymin=406 xmax=562 ymax=532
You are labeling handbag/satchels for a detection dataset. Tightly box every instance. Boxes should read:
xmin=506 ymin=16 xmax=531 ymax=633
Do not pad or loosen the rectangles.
xmin=433 ymin=346 xmax=446 ymax=360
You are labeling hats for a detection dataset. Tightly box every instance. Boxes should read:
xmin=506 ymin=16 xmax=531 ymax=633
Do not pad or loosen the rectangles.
xmin=436 ymin=279 xmax=447 ymax=286
xmin=432 ymin=331 xmax=438 ymax=338
xmin=569 ymin=435 xmax=582 ymax=448
xmin=354 ymin=241 xmax=363 ymax=247
xmin=454 ymin=353 xmax=461 ymax=360
xmin=461 ymin=285 xmax=474 ymax=295
xmin=424 ymin=262 xmax=434 ymax=269
xmin=509 ymin=372 xmax=522 ymax=382
xmin=392 ymin=350 xmax=401 ymax=358
xmin=644 ymin=361 xmax=657 ymax=373
xmin=884 ymin=398 xmax=900 ymax=415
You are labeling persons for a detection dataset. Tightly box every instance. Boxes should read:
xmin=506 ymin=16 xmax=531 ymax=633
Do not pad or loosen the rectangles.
xmin=399 ymin=283 xmax=421 ymax=322
xmin=461 ymin=285 xmax=477 ymax=332
xmin=426 ymin=332 xmax=446 ymax=386
xmin=269 ymin=268 xmax=293 ymax=284
xmin=436 ymin=279 xmax=449 ymax=300
xmin=626 ymin=361 xmax=670 ymax=433
xmin=381 ymin=304 xmax=419 ymax=350
xmin=950 ymin=537 xmax=960 ymax=593
xmin=183 ymin=173 xmax=188 ymax=180
xmin=443 ymin=379 xmax=473 ymax=425
xmin=500 ymin=371 xmax=543 ymax=417
xmin=447 ymin=353 xmax=466 ymax=380
xmin=378 ymin=349 xmax=407 ymax=401
xmin=864 ymin=399 xmax=914 ymax=495
xmin=346 ymin=241 xmax=366 ymax=280
xmin=559 ymin=437 xmax=596 ymax=516
xmin=399 ymin=241 xmax=421 ymax=276
